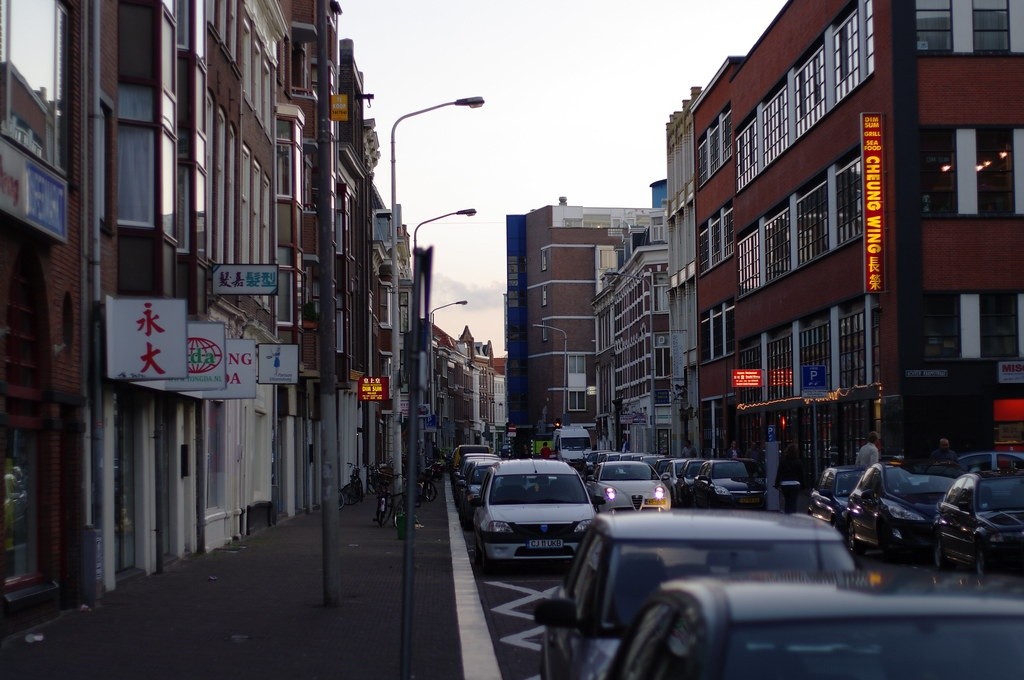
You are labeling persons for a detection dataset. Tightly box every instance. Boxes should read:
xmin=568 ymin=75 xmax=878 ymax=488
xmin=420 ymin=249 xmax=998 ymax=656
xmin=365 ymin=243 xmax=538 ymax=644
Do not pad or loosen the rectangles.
xmin=682 ymin=440 xmax=697 ymax=458
xmin=727 ymin=440 xmax=742 ymax=458
xmin=855 ymin=431 xmax=879 ymax=465
xmin=749 ymin=441 xmax=763 ymax=461
xmin=928 ymin=437 xmax=959 ymax=465
xmin=540 ymin=441 xmax=552 ymax=459
xmin=773 ymin=442 xmax=807 ymax=516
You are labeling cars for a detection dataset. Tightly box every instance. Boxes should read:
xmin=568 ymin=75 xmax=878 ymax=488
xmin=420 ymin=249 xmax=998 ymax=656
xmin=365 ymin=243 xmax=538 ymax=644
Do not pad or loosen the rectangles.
xmin=474 ymin=457 xmax=597 ymax=571
xmin=951 ymin=451 xmax=1024 ymax=469
xmin=606 ymin=569 xmax=1024 ymax=679
xmin=585 ymin=449 xmax=767 ymax=509
xmin=533 ymin=509 xmax=859 ymax=680
xmin=845 ymin=460 xmax=967 ymax=559
xmin=809 ymin=465 xmax=866 ymax=530
xmin=935 ymin=468 xmax=1023 ymax=571
xmin=581 ymin=460 xmax=672 ymax=511
xmin=453 ymin=443 xmax=503 ymax=528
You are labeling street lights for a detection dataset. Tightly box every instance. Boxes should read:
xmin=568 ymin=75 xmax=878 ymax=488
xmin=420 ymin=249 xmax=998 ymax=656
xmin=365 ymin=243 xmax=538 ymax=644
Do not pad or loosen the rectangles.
xmin=387 ymin=94 xmax=485 ymax=509
xmin=425 ymin=300 xmax=468 ymax=449
xmin=531 ymin=323 xmax=567 ymax=426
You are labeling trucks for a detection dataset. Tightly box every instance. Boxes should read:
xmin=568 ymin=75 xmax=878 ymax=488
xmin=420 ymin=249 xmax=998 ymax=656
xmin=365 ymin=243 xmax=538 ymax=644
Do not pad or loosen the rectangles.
xmin=552 ymin=424 xmax=593 ymax=463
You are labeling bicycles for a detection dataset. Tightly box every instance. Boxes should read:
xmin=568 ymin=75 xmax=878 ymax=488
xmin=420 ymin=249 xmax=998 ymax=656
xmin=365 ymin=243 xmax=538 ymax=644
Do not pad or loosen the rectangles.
xmin=338 ymin=455 xmax=446 ymax=528
